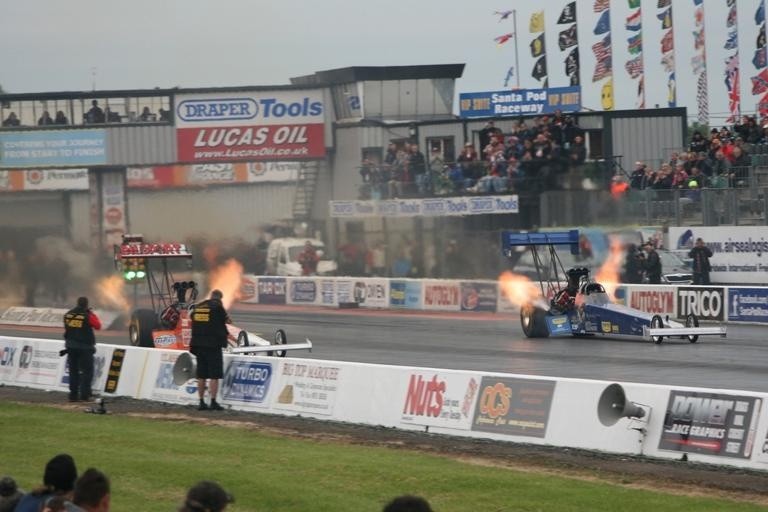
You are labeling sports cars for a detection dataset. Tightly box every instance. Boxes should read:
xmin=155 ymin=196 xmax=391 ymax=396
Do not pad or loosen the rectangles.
xmin=109 ymin=233 xmax=313 ymax=362
xmin=500 ymin=229 xmax=729 ymax=348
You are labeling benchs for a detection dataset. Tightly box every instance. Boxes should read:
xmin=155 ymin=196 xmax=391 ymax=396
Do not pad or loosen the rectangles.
xmin=355 ymin=124 xmax=629 ymax=196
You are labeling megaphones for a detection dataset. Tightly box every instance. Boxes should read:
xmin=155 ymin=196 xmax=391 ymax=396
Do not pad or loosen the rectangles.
xmin=173 ymin=353 xmax=197 ymax=385
xmin=597 ymin=383 xmax=646 ymax=427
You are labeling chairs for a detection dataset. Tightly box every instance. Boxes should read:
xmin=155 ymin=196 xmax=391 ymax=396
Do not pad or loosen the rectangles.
xmin=628 ymin=140 xmax=767 ymax=204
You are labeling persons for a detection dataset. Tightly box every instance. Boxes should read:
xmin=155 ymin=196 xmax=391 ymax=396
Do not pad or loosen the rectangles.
xmin=2 ymin=100 xmax=170 ymax=126
xmin=1 ymin=247 xmax=70 ymax=308
xmin=63 ymin=296 xmax=102 ymax=403
xmin=177 ymin=480 xmax=235 ymax=511
xmin=188 ymin=290 xmax=232 ymax=411
xmin=380 ymin=495 xmax=432 ymax=512
xmin=0 ymin=453 xmax=113 ymax=512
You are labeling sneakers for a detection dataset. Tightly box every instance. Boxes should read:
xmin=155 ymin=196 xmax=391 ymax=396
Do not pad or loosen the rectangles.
xmin=199 ymin=403 xmax=208 ymax=410
xmin=210 ymin=402 xmax=224 ymax=410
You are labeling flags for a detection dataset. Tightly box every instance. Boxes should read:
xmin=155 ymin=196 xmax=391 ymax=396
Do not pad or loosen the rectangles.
xmin=493 ymin=0 xmax=768 ymax=123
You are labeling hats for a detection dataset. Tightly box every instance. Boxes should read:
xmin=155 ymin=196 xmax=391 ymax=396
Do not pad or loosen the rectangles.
xmin=41 ymin=453 xmax=77 ymax=493
xmin=186 ymin=480 xmax=234 ymax=510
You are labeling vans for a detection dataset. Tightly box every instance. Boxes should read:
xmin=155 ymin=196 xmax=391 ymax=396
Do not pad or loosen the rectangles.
xmin=264 ymin=237 xmax=338 ymax=280
